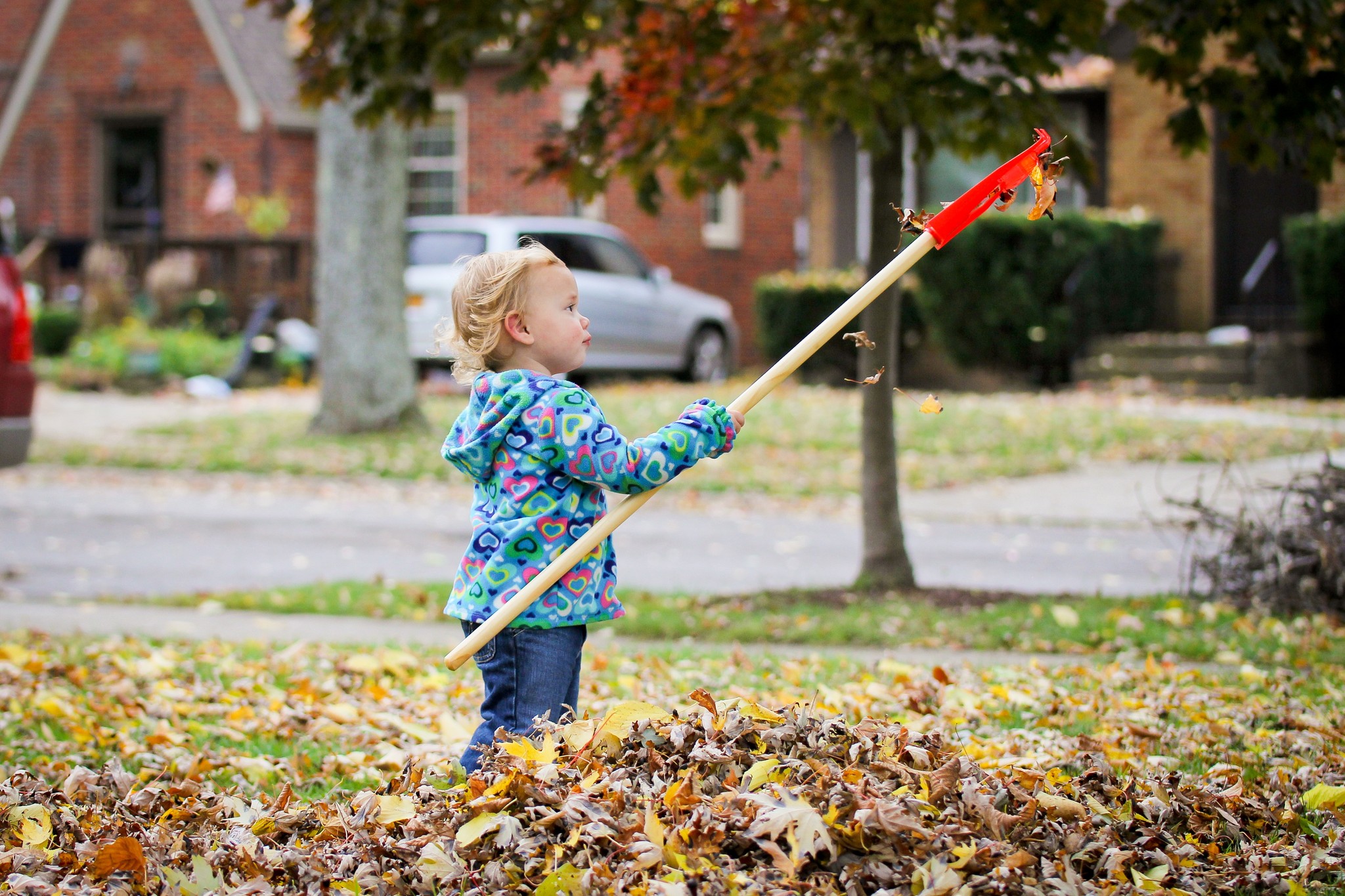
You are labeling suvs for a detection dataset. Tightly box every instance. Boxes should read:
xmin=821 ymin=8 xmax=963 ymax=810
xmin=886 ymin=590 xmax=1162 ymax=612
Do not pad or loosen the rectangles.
xmin=402 ymin=211 xmax=740 ymax=382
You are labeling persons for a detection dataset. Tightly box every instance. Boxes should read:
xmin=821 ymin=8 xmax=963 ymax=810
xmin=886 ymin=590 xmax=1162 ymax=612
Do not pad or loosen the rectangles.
xmin=0 ymin=195 xmax=320 ymax=401
xmin=437 ymin=238 xmax=747 ymax=779
